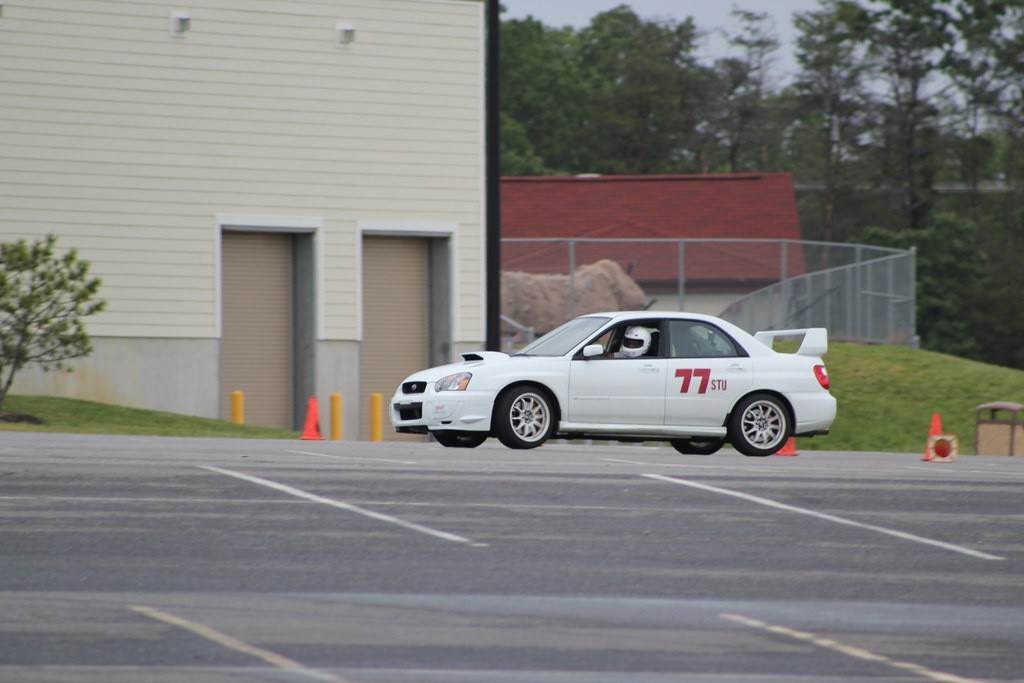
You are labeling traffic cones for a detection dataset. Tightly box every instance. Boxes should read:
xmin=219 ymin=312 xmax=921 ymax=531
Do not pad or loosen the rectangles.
xmin=919 ymin=414 xmax=959 ymax=462
xmin=298 ymin=397 xmax=326 ymax=440
xmin=776 ymin=436 xmax=799 ymax=456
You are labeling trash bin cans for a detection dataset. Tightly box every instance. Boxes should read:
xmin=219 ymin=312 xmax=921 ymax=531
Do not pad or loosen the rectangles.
xmin=976 ymin=402 xmax=1024 ymax=457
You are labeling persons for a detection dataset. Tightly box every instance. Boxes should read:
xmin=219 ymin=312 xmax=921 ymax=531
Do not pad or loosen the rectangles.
xmin=602 ymin=326 xmax=651 ymax=358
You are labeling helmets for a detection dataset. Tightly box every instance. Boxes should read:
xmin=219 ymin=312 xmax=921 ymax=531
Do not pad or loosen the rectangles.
xmin=619 ymin=327 xmax=651 ymax=357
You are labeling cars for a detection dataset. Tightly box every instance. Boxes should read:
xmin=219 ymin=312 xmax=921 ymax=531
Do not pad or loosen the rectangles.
xmin=388 ymin=311 xmax=838 ymax=457
